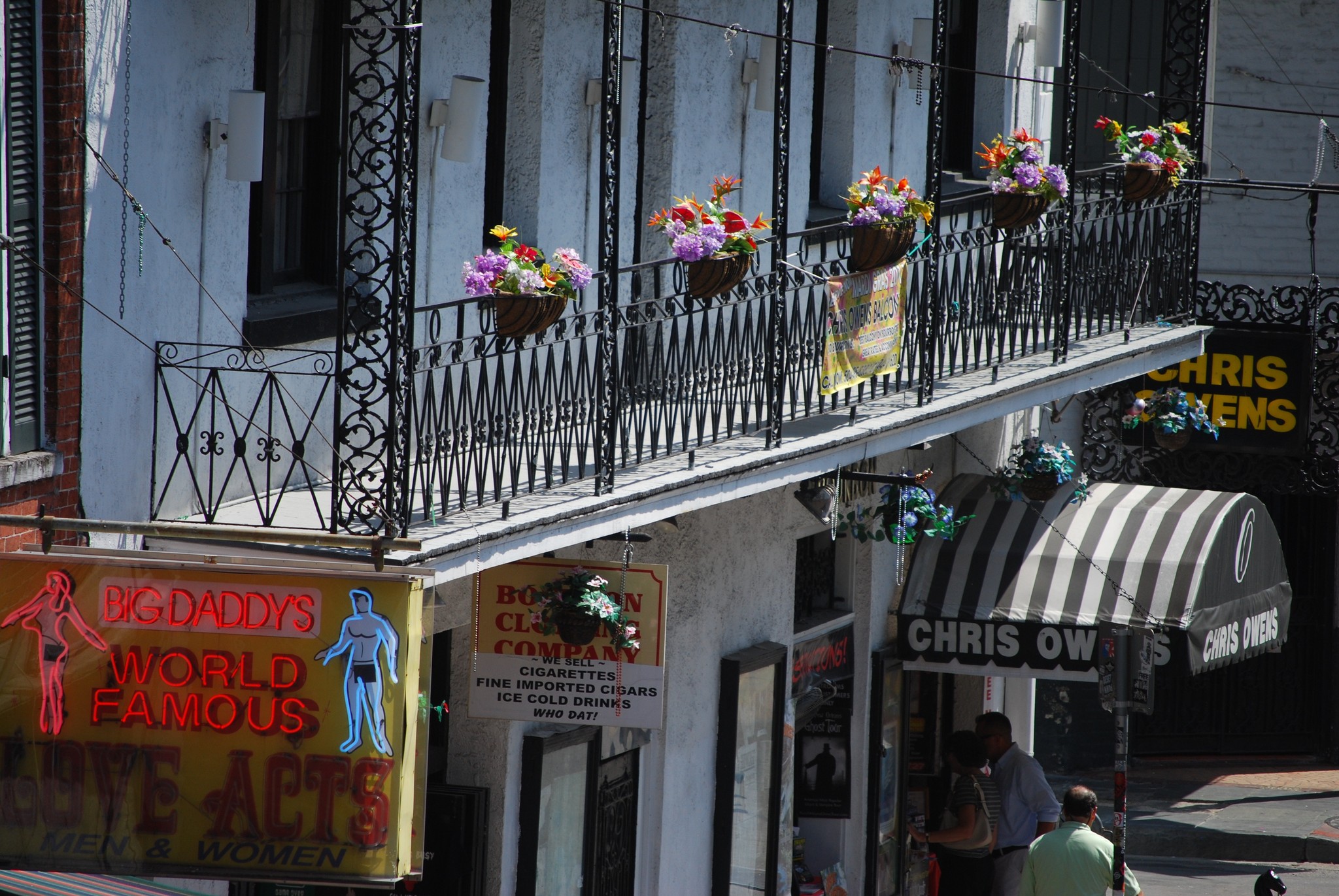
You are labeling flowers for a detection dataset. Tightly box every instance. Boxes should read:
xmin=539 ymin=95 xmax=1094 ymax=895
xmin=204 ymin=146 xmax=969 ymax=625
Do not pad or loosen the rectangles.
xmin=1093 ymin=115 xmax=1202 ymax=188
xmin=834 ymin=467 xmax=976 ymax=542
xmin=461 ymin=221 xmax=593 ymax=301
xmin=647 ymin=174 xmax=777 ymax=263
xmin=974 ymin=126 xmax=1070 ymax=203
xmin=1123 ymin=386 xmax=1226 ymax=441
xmin=837 ymin=166 xmax=934 ymax=229
xmin=418 ymin=691 xmax=449 ymax=724
xmin=509 ymin=564 xmax=640 ymax=651
xmin=985 ymin=426 xmax=1094 ymax=507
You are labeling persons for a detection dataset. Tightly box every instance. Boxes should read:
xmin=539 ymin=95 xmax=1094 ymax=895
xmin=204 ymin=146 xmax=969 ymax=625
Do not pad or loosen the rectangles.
xmin=907 ymin=730 xmax=1000 ymax=896
xmin=1018 ymin=785 xmax=1145 ymax=896
xmin=976 ymin=711 xmax=1060 ymax=896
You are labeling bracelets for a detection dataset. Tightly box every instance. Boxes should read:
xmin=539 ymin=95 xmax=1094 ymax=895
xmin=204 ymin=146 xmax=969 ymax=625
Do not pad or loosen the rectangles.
xmin=926 ymin=832 xmax=929 ymax=844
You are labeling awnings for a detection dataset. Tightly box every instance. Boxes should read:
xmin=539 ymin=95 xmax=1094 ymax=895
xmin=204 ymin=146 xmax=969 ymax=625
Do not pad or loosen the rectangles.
xmin=890 ymin=472 xmax=1292 ymax=681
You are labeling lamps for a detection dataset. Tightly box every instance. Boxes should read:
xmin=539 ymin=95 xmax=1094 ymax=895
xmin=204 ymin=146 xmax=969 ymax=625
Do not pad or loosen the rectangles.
xmin=898 ymin=17 xmax=941 ymax=89
xmin=585 ymin=57 xmax=635 ymax=137
xmin=422 ymin=586 xmax=447 ymax=609
xmin=429 ymin=75 xmax=486 ymax=163
xmin=1023 ymin=0 xmax=1065 ymax=66
xmin=208 ymin=89 xmax=265 ymax=181
xmin=744 ymin=34 xmax=777 ymax=111
xmin=1099 ymin=382 xmax=1146 ymax=416
xmin=794 ymin=481 xmax=837 ymax=525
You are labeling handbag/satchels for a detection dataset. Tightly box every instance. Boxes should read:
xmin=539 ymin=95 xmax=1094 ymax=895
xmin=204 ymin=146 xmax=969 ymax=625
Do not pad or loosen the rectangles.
xmin=939 ymin=774 xmax=992 ymax=849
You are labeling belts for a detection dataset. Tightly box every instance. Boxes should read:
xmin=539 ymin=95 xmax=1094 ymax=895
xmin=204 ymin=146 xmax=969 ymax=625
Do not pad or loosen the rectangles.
xmin=992 ymin=845 xmax=1028 ymax=859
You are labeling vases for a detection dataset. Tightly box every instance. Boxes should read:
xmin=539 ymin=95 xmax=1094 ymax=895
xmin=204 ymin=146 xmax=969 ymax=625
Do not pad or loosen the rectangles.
xmin=883 ymin=511 xmax=923 ymax=544
xmin=687 ymin=252 xmax=752 ymax=296
xmin=847 ymin=216 xmax=916 ymax=272
xmin=1022 ymin=476 xmax=1058 ymax=502
xmin=554 ymin=607 xmax=600 ymax=644
xmin=496 ymin=290 xmax=568 ymax=336
xmin=1125 ymin=162 xmax=1171 ymax=201
xmin=992 ymin=192 xmax=1050 ymax=228
xmin=1154 ymin=427 xmax=1192 ymax=451
